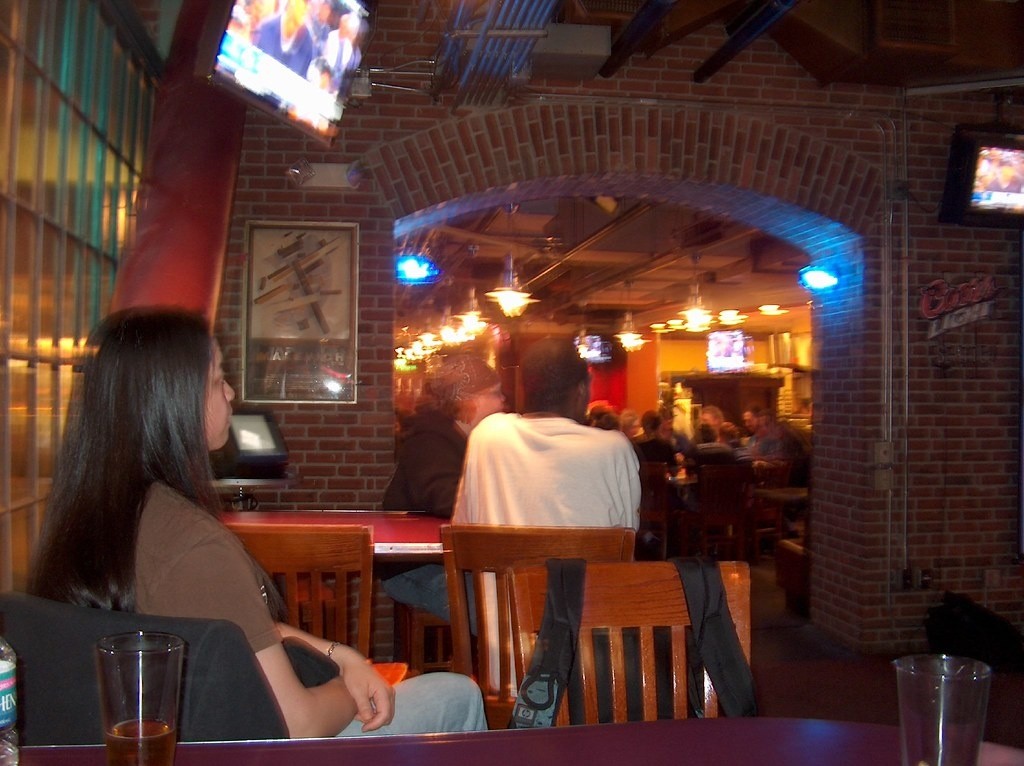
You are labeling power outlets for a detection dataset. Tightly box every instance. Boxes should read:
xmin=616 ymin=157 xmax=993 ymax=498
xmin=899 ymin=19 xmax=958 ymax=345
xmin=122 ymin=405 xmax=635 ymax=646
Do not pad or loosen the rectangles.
xmin=900 ymin=569 xmax=914 ymax=590
xmin=918 ymin=567 xmax=931 ymax=588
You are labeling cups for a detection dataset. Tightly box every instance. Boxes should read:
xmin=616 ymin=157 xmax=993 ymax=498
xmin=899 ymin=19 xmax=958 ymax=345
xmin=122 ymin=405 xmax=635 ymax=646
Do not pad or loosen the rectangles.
xmin=896 ymin=656 xmax=993 ymax=766
xmin=95 ymin=632 xmax=185 ymax=766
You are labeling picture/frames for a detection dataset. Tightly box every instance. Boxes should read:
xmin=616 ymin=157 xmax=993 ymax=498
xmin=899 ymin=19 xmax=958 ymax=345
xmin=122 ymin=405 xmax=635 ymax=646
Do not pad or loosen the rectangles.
xmin=241 ymin=220 xmax=361 ymax=405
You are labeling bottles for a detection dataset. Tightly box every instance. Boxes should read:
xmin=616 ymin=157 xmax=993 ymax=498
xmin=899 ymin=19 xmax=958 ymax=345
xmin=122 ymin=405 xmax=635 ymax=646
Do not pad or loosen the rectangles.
xmin=0 ymin=633 xmax=19 ymax=766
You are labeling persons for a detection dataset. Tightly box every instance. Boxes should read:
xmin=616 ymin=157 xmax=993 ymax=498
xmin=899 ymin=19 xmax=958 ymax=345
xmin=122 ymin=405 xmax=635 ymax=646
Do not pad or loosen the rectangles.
xmin=447 ymin=338 xmax=643 ymax=533
xmin=588 ymin=401 xmax=812 ymax=565
xmin=375 ymin=351 xmax=478 ymax=635
xmin=28 ymin=303 xmax=492 ymax=738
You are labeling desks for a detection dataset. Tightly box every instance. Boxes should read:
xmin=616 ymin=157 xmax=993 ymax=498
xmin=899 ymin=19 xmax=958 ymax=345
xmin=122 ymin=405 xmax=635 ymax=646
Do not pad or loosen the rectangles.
xmin=205 ymin=511 xmax=458 ymax=670
xmin=18 ymin=716 xmax=1024 ymax=766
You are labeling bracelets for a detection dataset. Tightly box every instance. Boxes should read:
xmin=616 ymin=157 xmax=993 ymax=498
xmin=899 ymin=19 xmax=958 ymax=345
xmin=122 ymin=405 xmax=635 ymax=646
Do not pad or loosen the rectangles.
xmin=328 ymin=642 xmax=340 ymax=657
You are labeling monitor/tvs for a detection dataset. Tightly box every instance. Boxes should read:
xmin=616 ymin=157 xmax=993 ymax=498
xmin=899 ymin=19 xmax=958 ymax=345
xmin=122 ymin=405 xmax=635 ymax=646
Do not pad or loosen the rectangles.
xmin=572 ymin=336 xmax=613 ymax=363
xmin=394 ymin=254 xmax=443 ymax=287
xmin=936 ymin=133 xmax=1024 ymax=230
xmin=194 ymin=0 xmax=378 ymax=149
xmin=705 ymin=329 xmax=746 ymax=371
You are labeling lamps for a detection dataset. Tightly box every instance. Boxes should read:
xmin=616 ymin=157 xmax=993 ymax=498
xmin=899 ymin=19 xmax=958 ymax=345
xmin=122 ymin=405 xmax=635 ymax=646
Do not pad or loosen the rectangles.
xmin=395 ymin=203 xmax=538 ymax=375
xmin=573 ymin=261 xmax=793 ymax=365
xmin=799 ymin=260 xmax=841 ymax=292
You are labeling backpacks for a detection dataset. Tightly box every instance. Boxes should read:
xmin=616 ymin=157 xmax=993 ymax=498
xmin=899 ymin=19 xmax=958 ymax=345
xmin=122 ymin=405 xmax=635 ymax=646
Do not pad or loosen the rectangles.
xmin=507 ymin=555 xmax=756 ymax=728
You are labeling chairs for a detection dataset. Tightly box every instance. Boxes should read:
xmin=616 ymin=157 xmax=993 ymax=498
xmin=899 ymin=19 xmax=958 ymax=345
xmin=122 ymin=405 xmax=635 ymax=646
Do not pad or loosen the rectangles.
xmin=0 ymin=456 xmax=798 ymax=740
